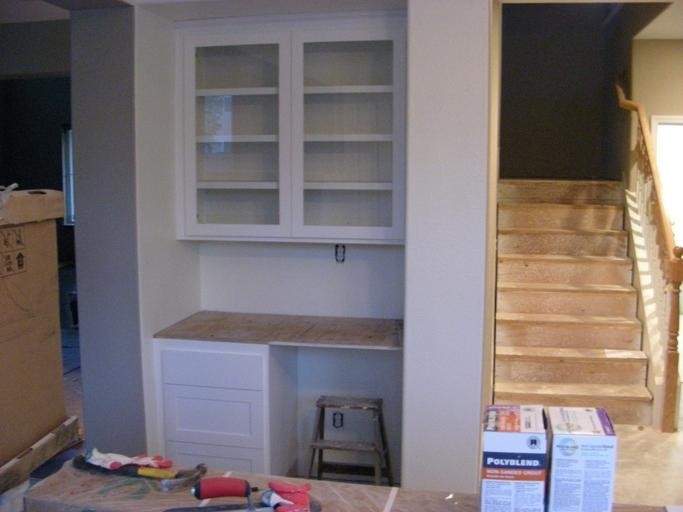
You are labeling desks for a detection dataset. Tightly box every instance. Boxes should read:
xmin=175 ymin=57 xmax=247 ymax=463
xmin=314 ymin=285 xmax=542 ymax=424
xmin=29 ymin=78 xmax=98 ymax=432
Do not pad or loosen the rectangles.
xmin=0 ymin=457 xmax=640 ymax=512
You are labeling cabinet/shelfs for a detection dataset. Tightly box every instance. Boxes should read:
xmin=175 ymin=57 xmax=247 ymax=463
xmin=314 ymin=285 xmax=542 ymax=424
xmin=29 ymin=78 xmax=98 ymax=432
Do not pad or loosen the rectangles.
xmin=172 ymin=10 xmax=405 ymax=248
xmin=152 ymin=309 xmax=403 ymax=476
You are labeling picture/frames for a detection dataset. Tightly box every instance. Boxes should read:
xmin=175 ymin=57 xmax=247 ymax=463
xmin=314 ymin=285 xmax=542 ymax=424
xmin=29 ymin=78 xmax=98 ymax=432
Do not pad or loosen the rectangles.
xmin=650 ymin=116 xmax=683 ymax=159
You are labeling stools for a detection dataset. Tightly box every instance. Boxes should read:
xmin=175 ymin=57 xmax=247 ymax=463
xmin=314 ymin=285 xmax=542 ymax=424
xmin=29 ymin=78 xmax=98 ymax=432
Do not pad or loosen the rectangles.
xmin=299 ymin=396 xmax=393 ymax=487
